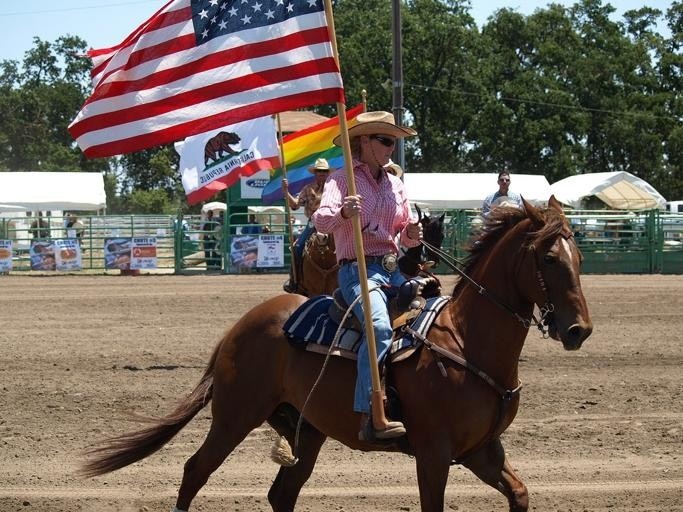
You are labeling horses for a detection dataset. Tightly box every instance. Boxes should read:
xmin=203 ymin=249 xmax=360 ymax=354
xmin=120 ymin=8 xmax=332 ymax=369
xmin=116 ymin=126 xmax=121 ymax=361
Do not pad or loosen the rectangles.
xmin=82 ymin=197 xmax=595 ymax=512
xmin=398 ymin=210 xmax=447 ymax=280
xmin=296 ymin=226 xmax=339 ymax=300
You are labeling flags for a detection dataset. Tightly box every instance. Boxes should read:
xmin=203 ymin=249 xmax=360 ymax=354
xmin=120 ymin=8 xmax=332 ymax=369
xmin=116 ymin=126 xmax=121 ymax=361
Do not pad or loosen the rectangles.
xmin=68 ymin=0 xmax=346 ymax=159
xmin=260 ymin=103 xmax=364 ymax=206
xmin=175 ymin=106 xmax=281 ymax=207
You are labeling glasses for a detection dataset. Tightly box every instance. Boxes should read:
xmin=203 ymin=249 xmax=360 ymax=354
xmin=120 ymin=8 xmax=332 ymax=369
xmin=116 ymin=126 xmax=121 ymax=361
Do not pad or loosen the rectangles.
xmin=372 ymin=136 xmax=397 ymax=147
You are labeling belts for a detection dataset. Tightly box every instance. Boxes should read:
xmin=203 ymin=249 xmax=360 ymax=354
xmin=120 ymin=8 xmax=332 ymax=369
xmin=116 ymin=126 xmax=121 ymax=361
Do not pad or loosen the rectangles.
xmin=341 ymin=255 xmax=396 ymax=273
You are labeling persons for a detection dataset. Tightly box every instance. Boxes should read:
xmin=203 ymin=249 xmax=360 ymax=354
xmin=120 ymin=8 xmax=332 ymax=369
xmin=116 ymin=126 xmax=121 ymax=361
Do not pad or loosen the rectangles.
xmin=241 ymin=215 xmax=263 ymax=234
xmin=201 ymin=210 xmax=217 ymax=270
xmin=312 ymin=111 xmax=423 ymax=443
xmin=29 ymin=212 xmax=49 ymax=238
xmin=482 ymin=172 xmax=525 ymax=225
xmin=282 ymin=157 xmax=331 ymax=293
xmin=64 ymin=212 xmax=84 ymax=247
xmin=572 ymin=210 xmax=584 ymax=239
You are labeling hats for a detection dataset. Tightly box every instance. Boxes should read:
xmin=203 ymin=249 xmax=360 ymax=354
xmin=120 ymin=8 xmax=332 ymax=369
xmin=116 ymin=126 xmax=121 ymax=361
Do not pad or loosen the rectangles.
xmin=382 ymin=158 xmax=403 ymax=178
xmin=333 ymin=111 xmax=418 ymax=146
xmin=308 ymin=158 xmax=335 ymax=174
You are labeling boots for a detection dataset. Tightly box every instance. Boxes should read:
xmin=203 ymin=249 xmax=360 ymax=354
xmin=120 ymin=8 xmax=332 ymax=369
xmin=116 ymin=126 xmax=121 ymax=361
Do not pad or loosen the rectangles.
xmin=357 ymin=412 xmax=406 ymax=440
xmin=284 ymin=257 xmax=300 ymax=293
